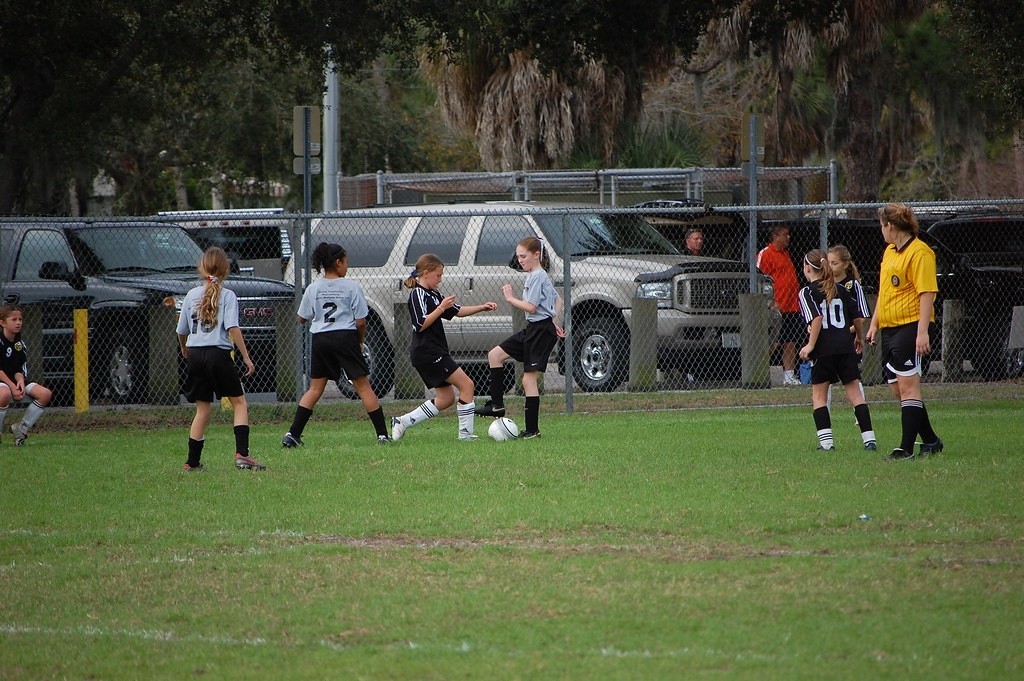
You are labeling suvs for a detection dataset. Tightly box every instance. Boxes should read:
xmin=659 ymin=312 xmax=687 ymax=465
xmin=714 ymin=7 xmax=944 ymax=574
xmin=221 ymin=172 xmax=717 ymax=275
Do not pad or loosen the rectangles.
xmin=139 ymin=206 xmax=309 ymax=282
xmin=282 ymin=202 xmax=783 ymax=394
xmin=0 ymin=218 xmax=307 ymax=407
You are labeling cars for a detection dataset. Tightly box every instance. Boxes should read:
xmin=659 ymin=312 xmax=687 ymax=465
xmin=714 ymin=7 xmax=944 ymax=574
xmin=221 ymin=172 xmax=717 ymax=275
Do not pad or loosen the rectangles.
xmin=626 ymin=198 xmax=1024 ymax=379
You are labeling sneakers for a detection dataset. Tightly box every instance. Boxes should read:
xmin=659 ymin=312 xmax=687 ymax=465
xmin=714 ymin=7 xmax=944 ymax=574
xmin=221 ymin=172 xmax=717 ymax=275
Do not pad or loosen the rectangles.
xmin=282 ymin=432 xmax=304 ymax=448
xmin=890 ymin=448 xmax=914 ymax=460
xmin=234 ymin=453 xmax=266 ymax=470
xmin=475 ymin=400 xmax=505 ymax=418
xmin=519 ymin=430 xmax=541 ymax=439
xmin=458 ymin=433 xmax=477 ymax=439
xmin=11 ymin=423 xmax=27 ymax=446
xmin=919 ymin=438 xmax=943 ymax=456
xmin=391 ymin=416 xmax=406 ymax=441
xmin=183 ymin=462 xmax=204 ymax=471
xmin=378 ymin=434 xmax=389 ymax=444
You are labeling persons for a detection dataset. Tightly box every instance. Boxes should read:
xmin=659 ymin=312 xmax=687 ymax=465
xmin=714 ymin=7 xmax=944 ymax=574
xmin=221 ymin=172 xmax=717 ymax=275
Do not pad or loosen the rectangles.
xmin=680 ymin=228 xmax=703 ymax=256
xmin=282 ymin=241 xmax=392 ymax=445
xmin=474 ymin=237 xmax=566 ymax=439
xmin=390 ymin=254 xmax=498 ymax=440
xmin=797 ymin=249 xmax=877 ymax=451
xmin=0 ymin=304 xmax=52 ymax=446
xmin=865 ymin=203 xmax=944 ymax=461
xmin=754 ymin=225 xmax=803 ymax=386
xmin=825 ymin=244 xmax=872 ymax=425
xmin=175 ymin=246 xmax=267 ymax=471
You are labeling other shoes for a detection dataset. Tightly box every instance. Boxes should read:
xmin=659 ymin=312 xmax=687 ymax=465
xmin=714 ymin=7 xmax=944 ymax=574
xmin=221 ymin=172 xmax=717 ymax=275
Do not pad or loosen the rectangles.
xmin=865 ymin=443 xmax=876 ymax=449
xmin=783 ymin=376 xmax=801 ymax=387
xmin=817 ymin=445 xmax=834 ymax=450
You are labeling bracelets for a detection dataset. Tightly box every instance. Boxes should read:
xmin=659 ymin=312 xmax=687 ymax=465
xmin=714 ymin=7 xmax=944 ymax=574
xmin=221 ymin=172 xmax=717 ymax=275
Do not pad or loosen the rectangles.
xmin=438 ymin=306 xmax=444 ymax=313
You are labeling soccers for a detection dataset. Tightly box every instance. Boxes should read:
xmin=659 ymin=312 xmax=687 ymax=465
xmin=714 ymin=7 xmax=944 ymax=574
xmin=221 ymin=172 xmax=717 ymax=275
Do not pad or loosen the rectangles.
xmin=487 ymin=417 xmax=519 ymax=442
